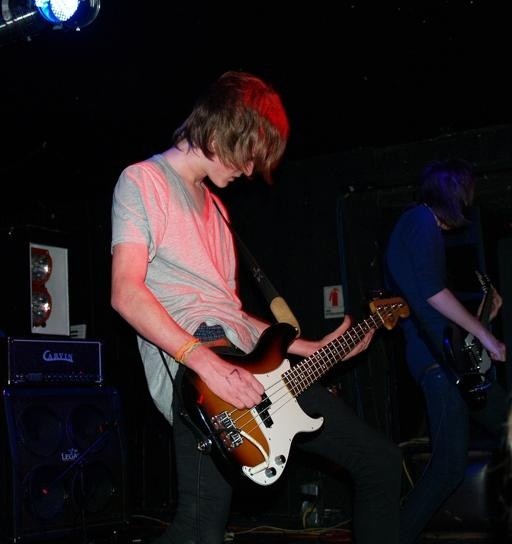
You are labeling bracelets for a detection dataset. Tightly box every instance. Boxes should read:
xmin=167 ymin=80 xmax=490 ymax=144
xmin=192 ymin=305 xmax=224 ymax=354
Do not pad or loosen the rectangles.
xmin=174 ymin=338 xmax=202 ymax=364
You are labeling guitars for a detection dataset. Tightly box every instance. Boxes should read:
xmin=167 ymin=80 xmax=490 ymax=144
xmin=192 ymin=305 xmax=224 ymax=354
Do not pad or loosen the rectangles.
xmin=178 ymin=297 xmax=409 ymax=488
xmin=446 ymin=271 xmax=497 ymax=396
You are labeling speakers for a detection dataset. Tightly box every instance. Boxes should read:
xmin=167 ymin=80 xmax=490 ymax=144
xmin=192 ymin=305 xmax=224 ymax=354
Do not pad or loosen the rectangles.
xmin=1 ymin=385 xmax=130 ymax=543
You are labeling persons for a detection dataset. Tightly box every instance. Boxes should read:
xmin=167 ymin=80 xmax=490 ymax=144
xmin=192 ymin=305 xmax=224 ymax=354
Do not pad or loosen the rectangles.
xmin=386 ymin=157 xmax=507 ymax=544
xmin=109 ymin=69 xmax=403 ymax=544
